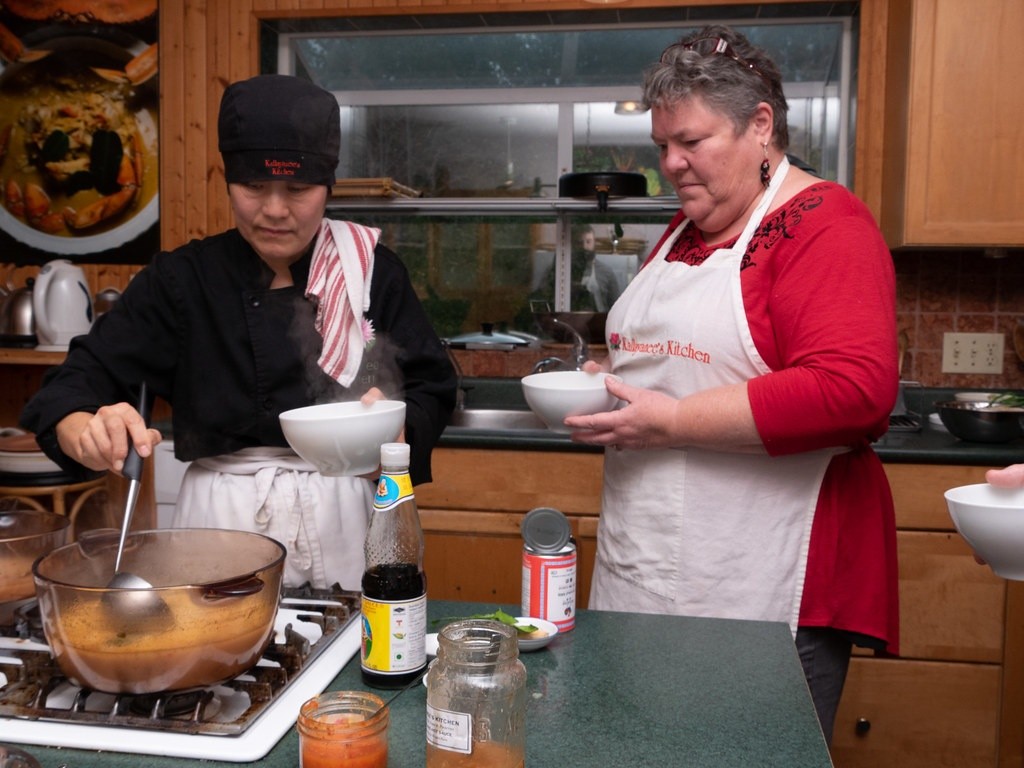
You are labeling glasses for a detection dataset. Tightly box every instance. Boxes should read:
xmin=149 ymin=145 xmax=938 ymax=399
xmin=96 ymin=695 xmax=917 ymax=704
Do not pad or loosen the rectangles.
xmin=659 ymin=36 xmax=773 ymax=97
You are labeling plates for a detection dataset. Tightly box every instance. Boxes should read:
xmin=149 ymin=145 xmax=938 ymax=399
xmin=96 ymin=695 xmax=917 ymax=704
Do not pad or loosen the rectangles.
xmin=0 ymin=40 xmax=159 ymax=255
xmin=426 ymin=617 xmax=560 ymax=657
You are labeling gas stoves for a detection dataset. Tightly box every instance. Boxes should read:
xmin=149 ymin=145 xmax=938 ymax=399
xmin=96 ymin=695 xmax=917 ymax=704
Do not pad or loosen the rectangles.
xmin=0 ymin=581 xmax=361 ymax=762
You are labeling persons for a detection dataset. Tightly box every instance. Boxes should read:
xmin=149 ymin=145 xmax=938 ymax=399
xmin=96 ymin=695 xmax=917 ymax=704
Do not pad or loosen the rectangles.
xmin=564 ymin=23 xmax=900 ymax=747
xmin=540 ymin=222 xmax=621 ymax=314
xmin=22 ymin=75 xmax=459 ymax=594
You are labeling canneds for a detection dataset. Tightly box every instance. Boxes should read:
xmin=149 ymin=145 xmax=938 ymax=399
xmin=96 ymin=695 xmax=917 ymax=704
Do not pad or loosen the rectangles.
xmin=296 ymin=691 xmax=390 ymax=768
xmin=521 ymin=506 xmax=577 ymax=633
xmin=425 ymin=619 xmax=528 ymax=768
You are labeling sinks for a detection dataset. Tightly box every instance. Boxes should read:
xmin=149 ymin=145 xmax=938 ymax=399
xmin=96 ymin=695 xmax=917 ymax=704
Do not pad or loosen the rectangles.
xmin=444 ymin=408 xmax=550 ymax=436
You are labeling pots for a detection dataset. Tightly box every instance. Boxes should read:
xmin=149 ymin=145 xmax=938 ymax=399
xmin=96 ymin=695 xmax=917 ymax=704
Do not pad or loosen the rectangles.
xmin=558 ymin=170 xmax=647 ymax=215
xmin=31 ymin=528 xmax=286 ymax=695
xmin=530 ymin=311 xmax=608 ymax=342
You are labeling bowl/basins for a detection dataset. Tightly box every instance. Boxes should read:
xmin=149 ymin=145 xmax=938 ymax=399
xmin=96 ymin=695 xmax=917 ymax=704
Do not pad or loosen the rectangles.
xmin=521 ymin=371 xmax=622 ymax=432
xmin=0 ymin=511 xmax=71 ymax=591
xmin=277 ymin=398 xmax=408 ymax=477
xmin=932 ymin=402 xmax=1024 ymax=446
xmin=942 ymin=482 xmax=1024 ymax=582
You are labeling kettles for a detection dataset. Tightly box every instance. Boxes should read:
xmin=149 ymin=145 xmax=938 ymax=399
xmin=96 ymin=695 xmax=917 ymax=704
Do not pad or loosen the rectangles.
xmin=0 ymin=258 xmax=94 ymax=350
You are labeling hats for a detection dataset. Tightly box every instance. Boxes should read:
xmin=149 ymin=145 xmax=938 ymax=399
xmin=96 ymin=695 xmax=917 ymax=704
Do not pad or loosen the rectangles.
xmin=218 ymin=74 xmax=341 ymax=184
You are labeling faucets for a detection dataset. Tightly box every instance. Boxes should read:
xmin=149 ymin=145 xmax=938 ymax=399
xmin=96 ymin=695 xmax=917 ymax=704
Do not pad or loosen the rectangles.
xmin=527 ymin=317 xmax=589 ymax=375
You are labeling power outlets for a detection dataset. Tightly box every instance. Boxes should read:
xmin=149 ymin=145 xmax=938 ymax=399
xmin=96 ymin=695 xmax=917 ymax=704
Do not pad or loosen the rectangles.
xmin=942 ymin=332 xmax=1005 ymax=374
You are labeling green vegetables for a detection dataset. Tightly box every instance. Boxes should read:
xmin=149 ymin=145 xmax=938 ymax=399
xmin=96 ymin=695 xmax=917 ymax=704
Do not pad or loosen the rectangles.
xmin=431 ymin=608 xmax=538 ymax=632
xmin=41 ymin=126 xmax=123 ymax=195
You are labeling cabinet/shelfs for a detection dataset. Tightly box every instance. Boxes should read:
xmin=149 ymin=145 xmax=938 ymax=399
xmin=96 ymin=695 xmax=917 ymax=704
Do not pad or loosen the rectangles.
xmin=878 ymin=0 xmax=1024 ymax=248
xmin=410 ymin=449 xmax=1024 ymax=768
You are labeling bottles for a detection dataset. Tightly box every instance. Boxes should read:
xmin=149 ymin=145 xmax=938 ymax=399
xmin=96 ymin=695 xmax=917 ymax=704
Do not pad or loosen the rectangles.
xmin=360 ymin=442 xmax=427 ymax=678
xmin=426 ymin=618 xmax=525 ymax=768
xmin=291 ymin=691 xmax=390 ymax=763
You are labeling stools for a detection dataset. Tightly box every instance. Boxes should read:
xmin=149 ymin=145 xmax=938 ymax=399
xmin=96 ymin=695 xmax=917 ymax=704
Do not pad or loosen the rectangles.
xmin=0 ymin=470 xmax=118 ymax=546
